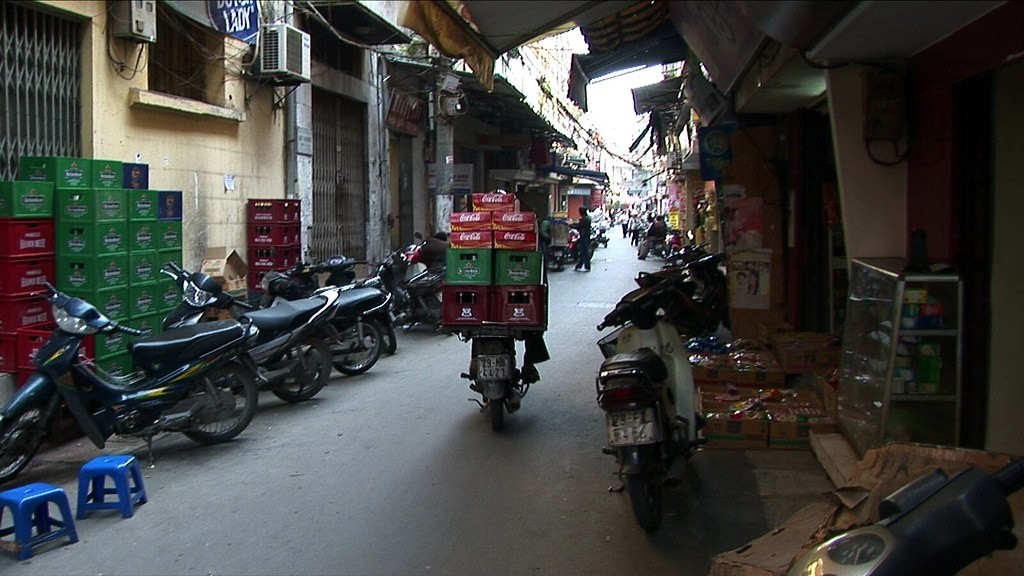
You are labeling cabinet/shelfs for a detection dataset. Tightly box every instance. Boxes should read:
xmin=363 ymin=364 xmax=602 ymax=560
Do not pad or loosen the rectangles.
xmin=834 ymin=256 xmax=962 ymax=453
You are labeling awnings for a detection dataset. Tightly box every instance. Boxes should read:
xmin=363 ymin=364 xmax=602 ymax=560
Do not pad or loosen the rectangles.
xmin=301 ymin=0 xmax=409 ymax=49
xmin=383 ymin=0 xmax=684 ymax=161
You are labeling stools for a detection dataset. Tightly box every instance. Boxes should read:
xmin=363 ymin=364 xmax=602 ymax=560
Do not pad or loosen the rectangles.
xmin=1 ymin=482 xmax=79 ymax=560
xmin=77 ymin=454 xmax=147 ymax=518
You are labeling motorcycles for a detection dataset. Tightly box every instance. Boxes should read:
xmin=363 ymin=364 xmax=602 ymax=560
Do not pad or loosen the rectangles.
xmin=252 ymin=256 xmax=393 ymax=375
xmin=368 ymin=240 xmax=446 ymax=335
xmin=0 ymin=281 xmax=268 ymax=483
xmin=160 ymin=260 xmax=345 ymax=404
xmin=461 ymin=268 xmax=541 ymax=428
xmin=307 ymin=254 xmax=399 ymax=357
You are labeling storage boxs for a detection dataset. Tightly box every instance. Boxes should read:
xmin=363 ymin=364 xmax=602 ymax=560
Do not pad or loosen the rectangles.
xmin=248 ymin=200 xmax=302 ymax=293
xmin=678 ymin=330 xmax=840 ymax=449
xmin=0 ymin=156 xmax=182 ymax=390
xmin=441 ymin=195 xmax=547 ymax=331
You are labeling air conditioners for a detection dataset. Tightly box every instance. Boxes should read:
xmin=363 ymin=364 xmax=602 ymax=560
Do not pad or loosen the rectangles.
xmin=260 ymin=22 xmax=312 ymax=83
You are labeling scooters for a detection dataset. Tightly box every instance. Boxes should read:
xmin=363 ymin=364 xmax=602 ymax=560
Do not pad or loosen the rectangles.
xmin=634 ymin=231 xmax=731 ymax=336
xmin=545 ymin=216 xmax=607 ymax=273
xmin=774 ymin=453 xmax=1024 ymax=576
xmin=594 ymin=269 xmax=708 ymax=532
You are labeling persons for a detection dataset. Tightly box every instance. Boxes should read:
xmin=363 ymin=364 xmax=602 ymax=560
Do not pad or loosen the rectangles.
xmin=736 ymin=270 xmax=760 ymax=303
xmin=469 ymin=189 xmax=551 ymax=384
xmin=618 ymin=207 xmax=682 ymax=260
xmin=540 ymin=215 xmax=554 ymax=268
xmin=570 ymin=207 xmax=592 ymax=272
